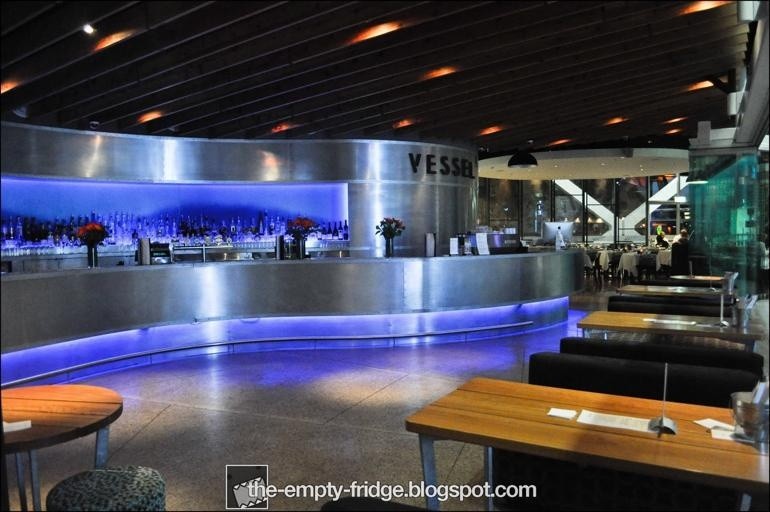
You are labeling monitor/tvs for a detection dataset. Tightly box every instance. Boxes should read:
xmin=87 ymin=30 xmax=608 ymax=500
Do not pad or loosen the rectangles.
xmin=543 ymin=222 xmax=574 ymax=250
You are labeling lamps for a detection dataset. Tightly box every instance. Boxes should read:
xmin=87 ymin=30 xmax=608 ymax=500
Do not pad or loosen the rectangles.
xmin=506 ymin=150 xmax=538 ymax=169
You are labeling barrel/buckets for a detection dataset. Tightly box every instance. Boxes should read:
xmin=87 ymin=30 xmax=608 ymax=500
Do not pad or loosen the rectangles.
xmin=721 ymin=278 xmax=734 ymax=291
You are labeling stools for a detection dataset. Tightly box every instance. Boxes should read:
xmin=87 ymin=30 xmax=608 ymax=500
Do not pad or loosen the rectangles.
xmin=44 ymin=463 xmax=165 ymax=511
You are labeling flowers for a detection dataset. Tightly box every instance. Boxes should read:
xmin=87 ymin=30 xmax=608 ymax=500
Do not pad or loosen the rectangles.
xmin=287 ymin=216 xmax=315 ymax=239
xmin=375 ymin=217 xmax=407 ymax=238
xmin=76 ymin=222 xmax=109 ymax=246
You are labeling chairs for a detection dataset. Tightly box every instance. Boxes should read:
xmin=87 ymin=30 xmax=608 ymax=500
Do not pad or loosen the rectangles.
xmin=570 ymin=241 xmax=685 ymax=288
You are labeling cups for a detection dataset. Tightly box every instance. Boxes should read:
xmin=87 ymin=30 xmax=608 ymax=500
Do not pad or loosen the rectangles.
xmin=722 ymin=278 xmax=735 ymax=292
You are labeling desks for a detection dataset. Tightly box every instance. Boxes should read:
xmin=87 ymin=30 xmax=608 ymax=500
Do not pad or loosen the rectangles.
xmin=0 ymin=385 xmax=124 ymax=511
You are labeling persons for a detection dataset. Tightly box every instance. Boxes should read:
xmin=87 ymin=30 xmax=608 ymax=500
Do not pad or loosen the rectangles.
xmin=656 ymin=235 xmax=669 ymax=248
xmin=655 ymin=231 xmax=669 ymax=248
xmin=677 ymin=227 xmax=690 ymax=246
xmin=757 ymin=230 xmax=770 ymax=301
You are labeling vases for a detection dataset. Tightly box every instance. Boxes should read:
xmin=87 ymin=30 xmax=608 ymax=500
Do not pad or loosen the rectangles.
xmin=88 ymin=246 xmax=98 ymax=268
xmin=296 ymin=238 xmax=308 ymax=259
xmin=385 ymin=237 xmax=393 ymax=257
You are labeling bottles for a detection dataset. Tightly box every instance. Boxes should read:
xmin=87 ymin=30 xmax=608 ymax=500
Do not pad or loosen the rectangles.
xmin=0 ymin=207 xmax=349 ymax=247
xmin=555 ymin=227 xmax=567 ymax=252
